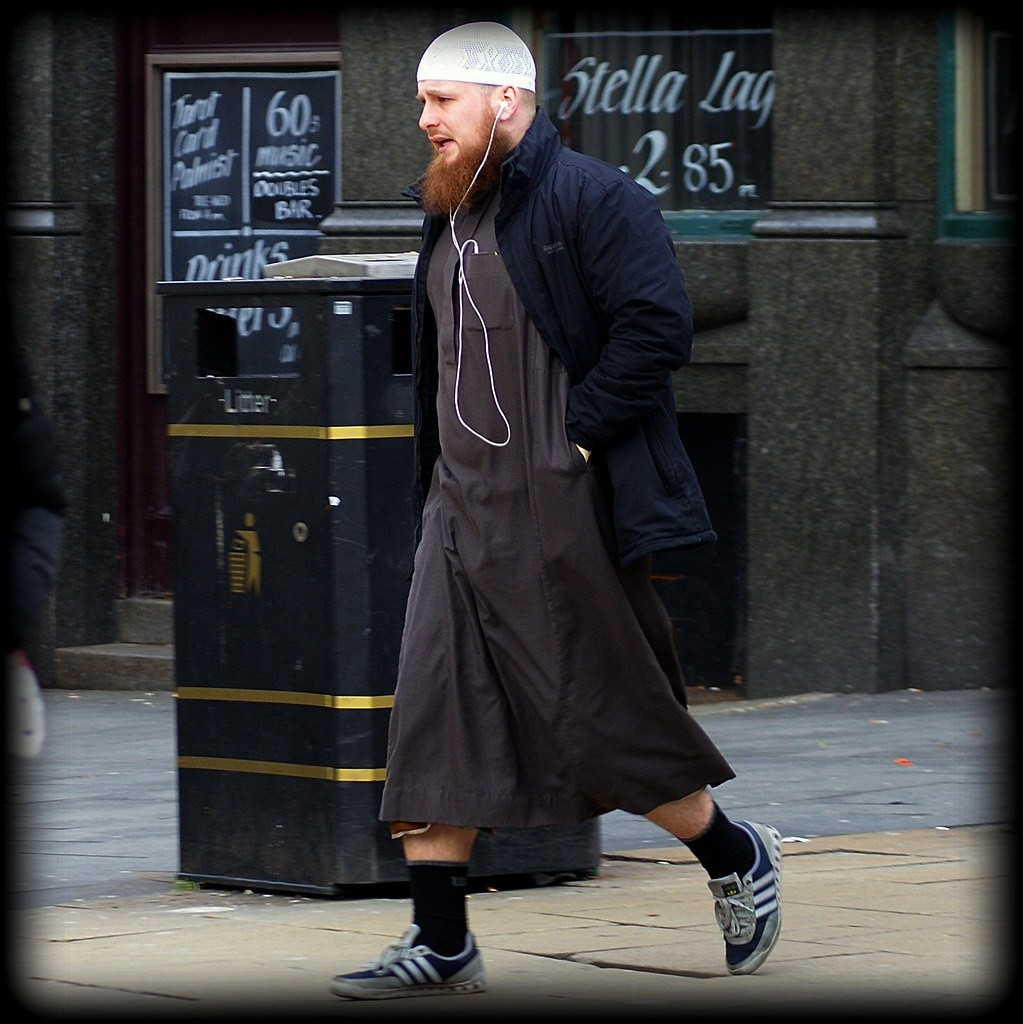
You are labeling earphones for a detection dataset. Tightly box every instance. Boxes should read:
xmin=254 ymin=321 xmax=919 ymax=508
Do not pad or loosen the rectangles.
xmin=497 ymin=100 xmax=509 ymax=119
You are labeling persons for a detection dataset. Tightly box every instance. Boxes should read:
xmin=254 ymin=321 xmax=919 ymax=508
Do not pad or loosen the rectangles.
xmin=328 ymin=21 xmax=783 ymax=999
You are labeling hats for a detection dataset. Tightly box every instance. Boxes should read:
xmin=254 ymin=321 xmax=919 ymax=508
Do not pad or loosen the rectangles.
xmin=417 ymin=21 xmax=536 ymax=92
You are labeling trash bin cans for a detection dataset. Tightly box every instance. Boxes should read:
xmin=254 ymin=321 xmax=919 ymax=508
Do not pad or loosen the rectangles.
xmin=153 ymin=251 xmax=608 ymax=897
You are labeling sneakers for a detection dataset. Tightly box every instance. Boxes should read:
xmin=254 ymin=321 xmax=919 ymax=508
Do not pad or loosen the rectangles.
xmin=707 ymin=821 xmax=782 ymax=975
xmin=331 ymin=923 xmax=485 ymax=999
